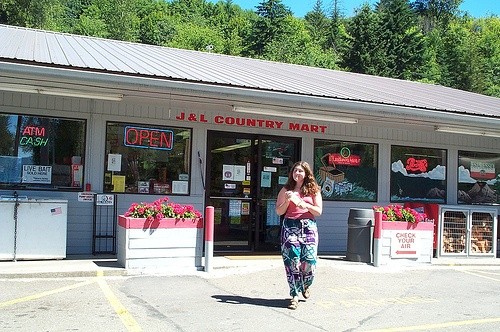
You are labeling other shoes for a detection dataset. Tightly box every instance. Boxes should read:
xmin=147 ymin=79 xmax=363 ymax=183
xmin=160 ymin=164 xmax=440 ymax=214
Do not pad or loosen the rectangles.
xmin=302 ymin=289 xmax=311 ymax=298
xmin=287 ymin=299 xmax=298 ymax=310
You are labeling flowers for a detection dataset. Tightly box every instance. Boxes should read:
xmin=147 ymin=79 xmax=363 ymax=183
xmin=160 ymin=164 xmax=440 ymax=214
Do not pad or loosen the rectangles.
xmin=125 ymin=197 xmax=203 ymax=221
xmin=372 ymin=203 xmax=427 ymax=224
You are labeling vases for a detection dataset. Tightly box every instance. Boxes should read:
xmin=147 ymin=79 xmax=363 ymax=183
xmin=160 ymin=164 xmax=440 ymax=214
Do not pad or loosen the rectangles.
xmin=118 ymin=215 xmax=205 ymax=268
xmin=382 ymin=221 xmax=435 ymax=263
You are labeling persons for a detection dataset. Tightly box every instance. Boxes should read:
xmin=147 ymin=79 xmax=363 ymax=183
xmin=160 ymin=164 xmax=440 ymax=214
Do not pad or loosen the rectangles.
xmin=275 ymin=161 xmax=323 ymax=310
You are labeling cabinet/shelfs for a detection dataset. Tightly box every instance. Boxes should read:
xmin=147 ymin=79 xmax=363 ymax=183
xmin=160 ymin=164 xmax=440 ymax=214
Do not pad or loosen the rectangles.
xmin=428 ymin=204 xmax=498 ymax=258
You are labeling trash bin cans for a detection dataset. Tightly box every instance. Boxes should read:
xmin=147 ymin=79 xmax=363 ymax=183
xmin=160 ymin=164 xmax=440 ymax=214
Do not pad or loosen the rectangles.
xmin=346 ymin=208 xmax=375 ymax=262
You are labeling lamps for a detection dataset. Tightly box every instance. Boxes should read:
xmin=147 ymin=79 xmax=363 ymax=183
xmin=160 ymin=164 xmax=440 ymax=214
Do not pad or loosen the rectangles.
xmin=434 ymin=127 xmax=500 ymax=137
xmin=232 ymin=106 xmax=358 ymax=124
xmin=0 ymin=82 xmax=125 ymax=102
